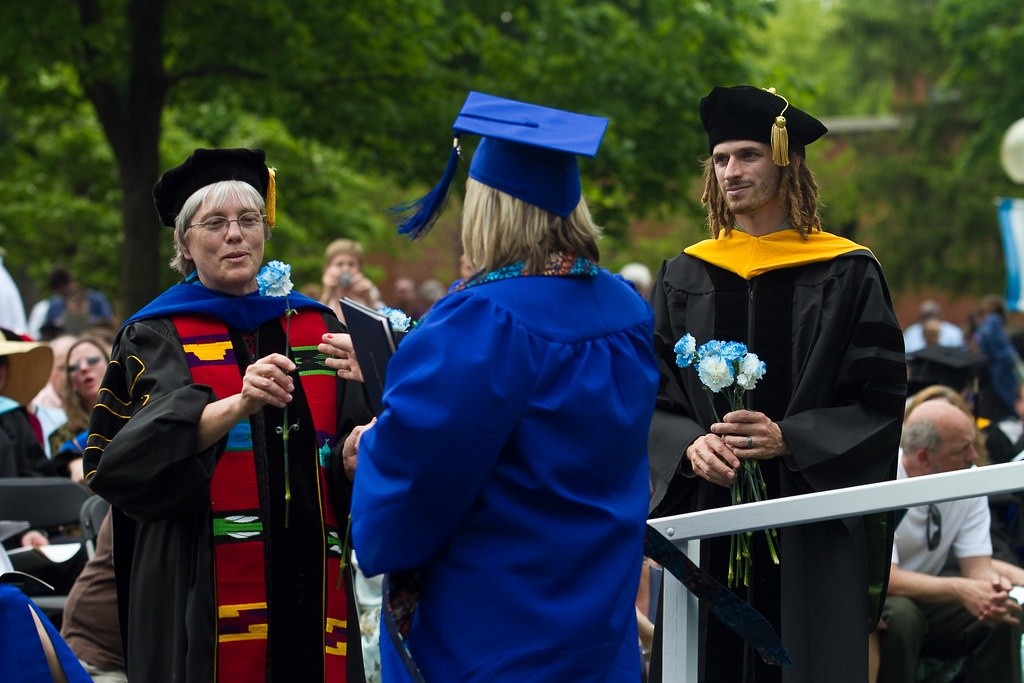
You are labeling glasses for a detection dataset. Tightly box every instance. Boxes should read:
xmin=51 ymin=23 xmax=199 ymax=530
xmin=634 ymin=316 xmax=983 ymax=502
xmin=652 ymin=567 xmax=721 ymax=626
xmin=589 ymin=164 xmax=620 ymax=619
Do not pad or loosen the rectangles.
xmin=68 ymin=355 xmax=104 ymax=373
xmin=185 ymin=212 xmax=269 ymax=233
xmin=926 ymin=503 xmax=942 ymax=551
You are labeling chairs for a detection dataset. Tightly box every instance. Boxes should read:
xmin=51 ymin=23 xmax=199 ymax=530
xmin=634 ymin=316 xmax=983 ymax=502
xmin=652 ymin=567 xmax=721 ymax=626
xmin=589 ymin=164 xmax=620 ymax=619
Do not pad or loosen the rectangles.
xmin=0 ymin=477 xmax=111 ymax=609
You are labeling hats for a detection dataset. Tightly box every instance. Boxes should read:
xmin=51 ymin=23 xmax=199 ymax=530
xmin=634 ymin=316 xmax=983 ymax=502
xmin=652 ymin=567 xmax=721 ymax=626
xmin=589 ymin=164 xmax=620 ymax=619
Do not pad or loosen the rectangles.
xmin=0 ymin=330 xmax=55 ymax=407
xmin=390 ymin=91 xmax=609 ymax=244
xmin=699 ymin=85 xmax=829 ymax=167
xmin=152 ymin=147 xmax=276 ymax=230
xmin=906 ymin=343 xmax=987 ymax=391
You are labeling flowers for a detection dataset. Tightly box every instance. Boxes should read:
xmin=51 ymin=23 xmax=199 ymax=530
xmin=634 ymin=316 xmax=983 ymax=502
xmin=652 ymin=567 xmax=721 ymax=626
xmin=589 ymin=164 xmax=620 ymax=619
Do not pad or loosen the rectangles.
xmin=674 ymin=332 xmax=780 ymax=590
xmin=374 ymin=307 xmax=411 ymax=344
xmin=256 ymin=261 xmax=300 ymax=528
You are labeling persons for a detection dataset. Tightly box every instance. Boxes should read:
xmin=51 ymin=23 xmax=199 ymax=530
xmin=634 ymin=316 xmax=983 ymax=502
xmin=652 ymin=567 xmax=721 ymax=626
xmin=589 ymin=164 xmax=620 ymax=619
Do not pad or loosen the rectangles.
xmin=82 ymin=149 xmax=376 ymax=683
xmin=648 ymin=86 xmax=909 ymax=683
xmin=299 ymin=240 xmax=656 ymax=329
xmin=0 ymin=255 xmax=129 ymax=683
xmin=351 ymin=92 xmax=660 ymax=683
xmin=875 ymin=293 xmax=1024 ymax=683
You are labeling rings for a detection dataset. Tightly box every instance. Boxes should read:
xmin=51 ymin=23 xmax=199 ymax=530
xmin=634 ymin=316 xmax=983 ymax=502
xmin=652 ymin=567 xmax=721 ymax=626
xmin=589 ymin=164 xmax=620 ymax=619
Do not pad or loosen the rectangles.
xmin=746 ymin=436 xmax=753 ymax=448
